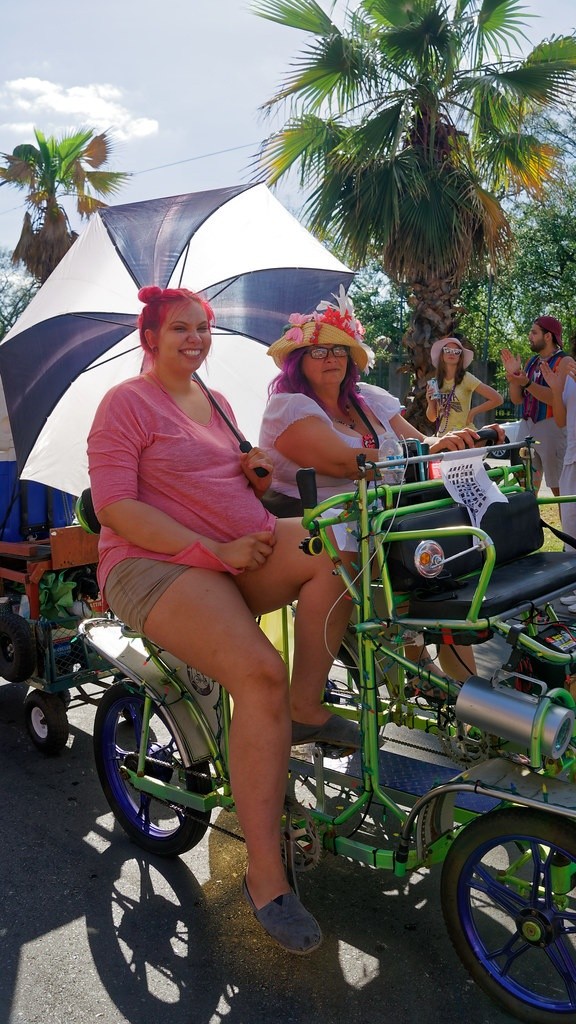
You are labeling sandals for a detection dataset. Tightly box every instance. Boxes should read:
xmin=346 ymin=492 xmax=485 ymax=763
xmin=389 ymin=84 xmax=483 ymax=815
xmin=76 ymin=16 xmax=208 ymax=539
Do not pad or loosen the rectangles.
xmin=403 ymin=657 xmax=464 ymax=704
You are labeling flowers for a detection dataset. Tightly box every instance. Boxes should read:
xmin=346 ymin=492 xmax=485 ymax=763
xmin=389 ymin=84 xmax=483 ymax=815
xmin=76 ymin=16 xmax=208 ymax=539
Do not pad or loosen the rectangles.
xmin=283 ymin=281 xmax=378 ymax=376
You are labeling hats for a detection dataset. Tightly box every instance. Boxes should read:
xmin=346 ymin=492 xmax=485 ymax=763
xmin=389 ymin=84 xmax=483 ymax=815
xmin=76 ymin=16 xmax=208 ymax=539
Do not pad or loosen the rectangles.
xmin=266 ymin=284 xmax=376 ymax=375
xmin=534 ymin=316 xmax=563 ymax=347
xmin=430 ymin=338 xmax=475 ymax=369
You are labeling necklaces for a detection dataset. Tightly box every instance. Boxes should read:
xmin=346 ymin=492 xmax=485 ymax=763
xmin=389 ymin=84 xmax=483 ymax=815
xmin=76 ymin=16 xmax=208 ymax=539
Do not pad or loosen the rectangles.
xmin=316 ymin=402 xmax=355 ymax=430
xmin=434 ymin=381 xmax=456 ymax=434
xmin=539 ymin=356 xmax=549 ymax=361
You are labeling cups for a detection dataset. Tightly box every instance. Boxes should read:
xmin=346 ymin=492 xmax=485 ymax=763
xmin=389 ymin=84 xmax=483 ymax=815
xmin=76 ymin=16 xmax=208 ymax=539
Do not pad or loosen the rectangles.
xmin=426 ymin=379 xmax=441 ymax=400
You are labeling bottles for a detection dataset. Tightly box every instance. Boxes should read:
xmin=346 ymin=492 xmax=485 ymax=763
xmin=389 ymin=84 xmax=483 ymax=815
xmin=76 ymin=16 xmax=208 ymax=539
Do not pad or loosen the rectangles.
xmin=378 ymin=433 xmax=404 ymax=483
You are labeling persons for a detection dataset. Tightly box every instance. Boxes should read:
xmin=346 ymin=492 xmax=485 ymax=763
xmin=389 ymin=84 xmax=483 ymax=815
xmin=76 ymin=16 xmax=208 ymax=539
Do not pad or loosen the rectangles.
xmin=500 ymin=316 xmax=567 ymax=522
xmin=89 ymin=279 xmax=356 ymax=953
xmin=553 ymin=355 xmax=576 ymax=611
xmin=256 ymin=280 xmax=508 ymax=706
xmin=424 ymin=337 xmax=506 ymax=440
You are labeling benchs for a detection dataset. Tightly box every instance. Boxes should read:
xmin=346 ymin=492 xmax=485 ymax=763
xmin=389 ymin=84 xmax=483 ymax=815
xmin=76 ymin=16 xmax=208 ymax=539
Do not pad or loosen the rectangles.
xmin=387 ymin=490 xmax=576 ymax=631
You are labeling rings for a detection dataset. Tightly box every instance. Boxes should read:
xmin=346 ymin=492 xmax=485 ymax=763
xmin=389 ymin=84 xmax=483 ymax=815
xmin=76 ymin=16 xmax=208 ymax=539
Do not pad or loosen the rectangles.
xmin=502 ymin=428 xmax=505 ymax=432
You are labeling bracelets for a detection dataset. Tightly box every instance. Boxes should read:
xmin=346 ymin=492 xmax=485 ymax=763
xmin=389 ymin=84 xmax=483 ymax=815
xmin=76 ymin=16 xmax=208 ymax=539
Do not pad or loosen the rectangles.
xmin=523 ymin=379 xmax=533 ymax=388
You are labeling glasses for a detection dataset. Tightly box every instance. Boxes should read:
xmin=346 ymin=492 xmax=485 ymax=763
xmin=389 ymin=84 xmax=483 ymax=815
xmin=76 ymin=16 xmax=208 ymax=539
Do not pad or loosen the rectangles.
xmin=442 ymin=346 xmax=463 ymax=357
xmin=303 ymin=344 xmax=349 ymax=360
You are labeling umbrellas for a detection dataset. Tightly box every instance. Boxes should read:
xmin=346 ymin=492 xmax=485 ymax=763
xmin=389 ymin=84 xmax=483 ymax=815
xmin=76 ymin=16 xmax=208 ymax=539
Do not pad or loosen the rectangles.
xmin=1 ymin=180 xmax=361 ymax=501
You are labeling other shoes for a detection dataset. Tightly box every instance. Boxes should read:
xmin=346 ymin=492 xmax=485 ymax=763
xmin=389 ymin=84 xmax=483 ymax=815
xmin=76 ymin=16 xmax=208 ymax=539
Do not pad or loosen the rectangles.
xmin=290 ymin=712 xmax=387 ymax=749
xmin=240 ymin=873 xmax=325 ymax=955
